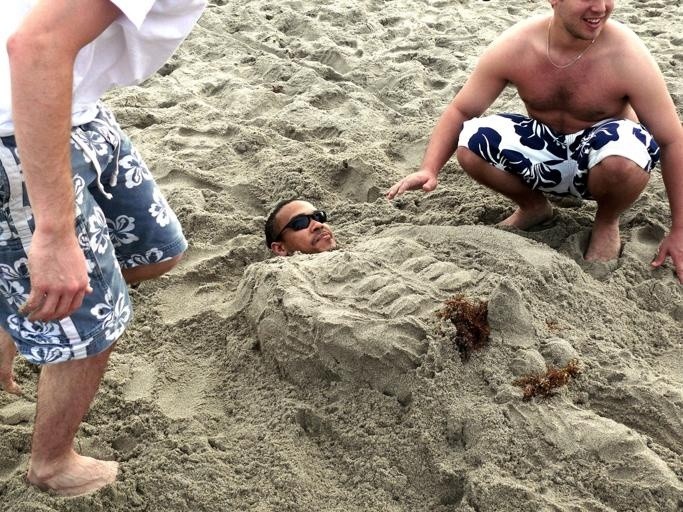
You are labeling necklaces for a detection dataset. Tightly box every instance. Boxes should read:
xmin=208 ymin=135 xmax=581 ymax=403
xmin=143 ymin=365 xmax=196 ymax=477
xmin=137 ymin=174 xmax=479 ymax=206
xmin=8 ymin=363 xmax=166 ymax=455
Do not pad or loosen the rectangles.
xmin=546 ymin=16 xmax=596 ymax=70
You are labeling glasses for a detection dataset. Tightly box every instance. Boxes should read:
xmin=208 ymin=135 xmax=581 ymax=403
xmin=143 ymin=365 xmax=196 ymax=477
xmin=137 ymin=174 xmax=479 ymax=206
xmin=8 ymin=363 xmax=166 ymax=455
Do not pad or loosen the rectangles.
xmin=274 ymin=210 xmax=328 ymax=243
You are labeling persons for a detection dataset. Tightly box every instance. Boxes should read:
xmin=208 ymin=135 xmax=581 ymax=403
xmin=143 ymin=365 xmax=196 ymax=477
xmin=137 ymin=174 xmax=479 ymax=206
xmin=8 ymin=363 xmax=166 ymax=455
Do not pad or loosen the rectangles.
xmin=260 ymin=198 xmax=339 ymax=259
xmin=383 ymin=1 xmax=683 ymax=288
xmin=1 ymin=2 xmax=212 ymax=499
